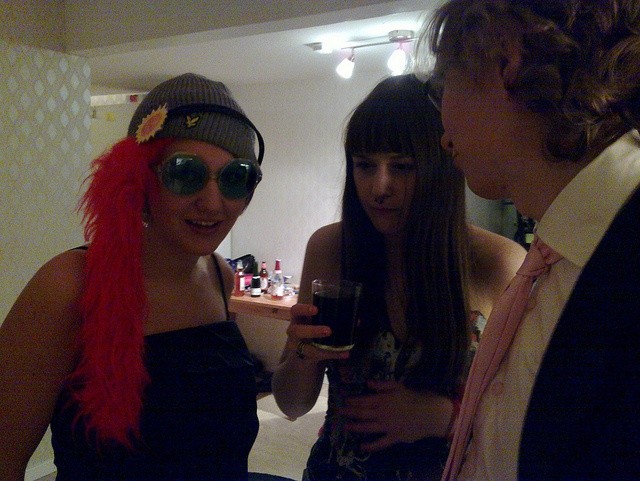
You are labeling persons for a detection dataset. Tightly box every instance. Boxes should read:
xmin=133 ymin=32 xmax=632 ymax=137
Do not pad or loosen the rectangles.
xmin=411 ymin=2 xmax=640 ymax=481
xmin=0 ymin=73 xmax=272 ymax=481
xmin=272 ymin=75 xmax=529 ymax=481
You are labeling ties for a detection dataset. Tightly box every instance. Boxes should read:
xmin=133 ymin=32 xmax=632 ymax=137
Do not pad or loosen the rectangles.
xmin=440 ymin=229 xmax=565 ymax=480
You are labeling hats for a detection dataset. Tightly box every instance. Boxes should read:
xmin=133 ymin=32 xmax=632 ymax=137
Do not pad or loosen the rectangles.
xmin=128 ymin=73 xmax=262 ymax=169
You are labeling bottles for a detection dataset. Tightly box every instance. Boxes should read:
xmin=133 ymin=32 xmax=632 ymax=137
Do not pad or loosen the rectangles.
xmin=272 ymin=259 xmax=284 ymax=298
xmin=252 ymin=262 xmax=261 ymax=297
xmin=261 ymin=261 xmax=268 ymax=292
xmin=234 ymin=261 xmax=245 ymax=296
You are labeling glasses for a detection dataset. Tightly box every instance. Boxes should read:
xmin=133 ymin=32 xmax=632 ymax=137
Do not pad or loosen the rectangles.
xmin=421 ymin=47 xmax=491 ymax=111
xmin=150 ymin=151 xmax=262 ymax=197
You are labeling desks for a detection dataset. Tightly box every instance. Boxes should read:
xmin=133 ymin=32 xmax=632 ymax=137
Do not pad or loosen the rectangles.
xmin=227 ymin=286 xmax=299 ymax=321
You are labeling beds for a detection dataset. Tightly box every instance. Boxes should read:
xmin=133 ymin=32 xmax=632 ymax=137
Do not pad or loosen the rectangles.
xmin=248 ymin=373 xmax=329 ymax=480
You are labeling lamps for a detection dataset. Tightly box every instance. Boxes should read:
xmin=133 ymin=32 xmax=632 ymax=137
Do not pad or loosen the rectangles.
xmin=385 ymin=30 xmax=414 ymax=76
xmin=336 ymin=47 xmax=358 ymax=80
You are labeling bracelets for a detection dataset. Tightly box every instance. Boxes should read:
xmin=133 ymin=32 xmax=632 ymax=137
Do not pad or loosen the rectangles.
xmin=445 ymin=397 xmax=461 ymax=442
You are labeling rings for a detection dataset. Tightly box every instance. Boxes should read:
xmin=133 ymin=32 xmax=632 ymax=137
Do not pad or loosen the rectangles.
xmin=295 ymin=340 xmax=306 ymax=360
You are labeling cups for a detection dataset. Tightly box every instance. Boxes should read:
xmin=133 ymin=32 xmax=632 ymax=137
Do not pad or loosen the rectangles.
xmin=310 ymin=279 xmax=361 ymax=352
